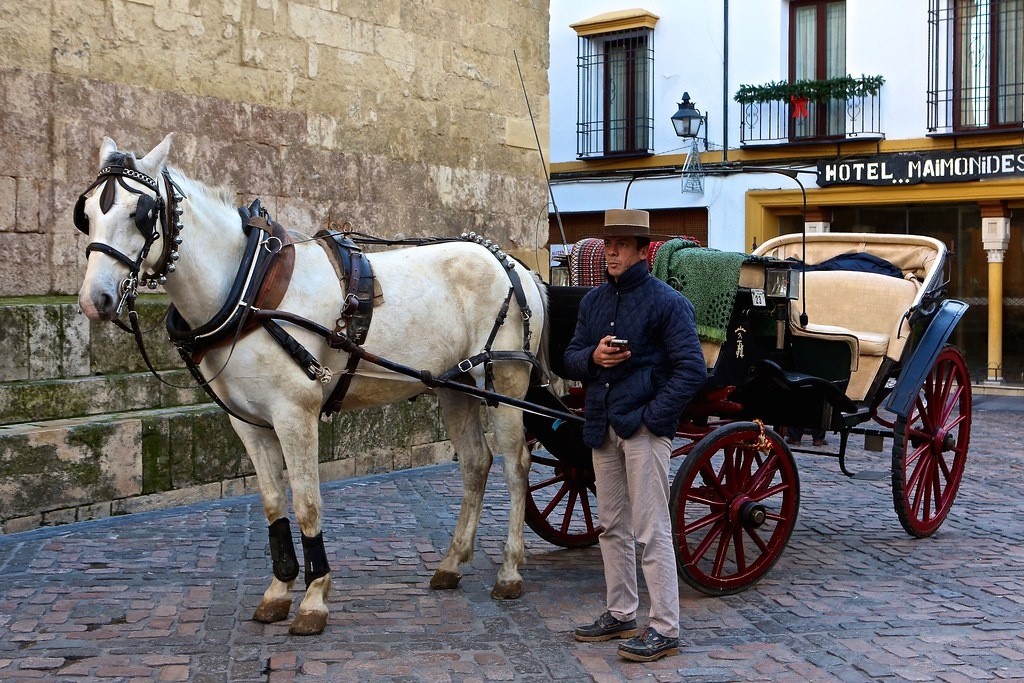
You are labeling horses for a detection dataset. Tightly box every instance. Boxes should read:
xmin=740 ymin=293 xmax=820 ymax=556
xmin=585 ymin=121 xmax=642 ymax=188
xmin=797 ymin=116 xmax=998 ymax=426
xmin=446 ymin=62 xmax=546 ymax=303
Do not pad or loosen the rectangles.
xmin=77 ymin=131 xmax=553 ymax=636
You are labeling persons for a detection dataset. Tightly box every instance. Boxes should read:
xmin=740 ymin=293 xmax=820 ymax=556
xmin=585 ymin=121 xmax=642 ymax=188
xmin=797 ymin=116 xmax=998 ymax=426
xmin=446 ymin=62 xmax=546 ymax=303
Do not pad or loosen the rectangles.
xmin=563 ymin=208 xmax=708 ymax=662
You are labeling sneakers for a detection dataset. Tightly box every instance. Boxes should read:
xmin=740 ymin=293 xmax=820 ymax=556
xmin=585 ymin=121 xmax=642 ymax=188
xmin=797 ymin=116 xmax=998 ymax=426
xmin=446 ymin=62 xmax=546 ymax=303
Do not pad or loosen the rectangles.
xmin=574 ymin=610 xmax=638 ymax=642
xmin=618 ymin=624 xmax=679 ymax=662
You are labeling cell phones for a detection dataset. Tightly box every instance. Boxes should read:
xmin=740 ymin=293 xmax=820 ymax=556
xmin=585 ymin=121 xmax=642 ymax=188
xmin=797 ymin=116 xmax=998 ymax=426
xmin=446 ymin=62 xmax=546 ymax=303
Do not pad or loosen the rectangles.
xmin=610 ymin=339 xmax=629 ymax=354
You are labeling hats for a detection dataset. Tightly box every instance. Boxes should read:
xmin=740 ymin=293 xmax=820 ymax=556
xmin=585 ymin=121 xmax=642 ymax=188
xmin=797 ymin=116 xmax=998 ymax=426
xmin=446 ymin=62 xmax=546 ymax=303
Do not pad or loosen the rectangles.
xmin=595 ymin=209 xmax=661 ymax=242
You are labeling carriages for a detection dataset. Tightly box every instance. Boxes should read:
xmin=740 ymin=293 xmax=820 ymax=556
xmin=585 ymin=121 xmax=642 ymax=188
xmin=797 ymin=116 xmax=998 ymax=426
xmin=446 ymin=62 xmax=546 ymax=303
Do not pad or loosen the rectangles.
xmin=72 ymin=128 xmax=974 ymax=636
